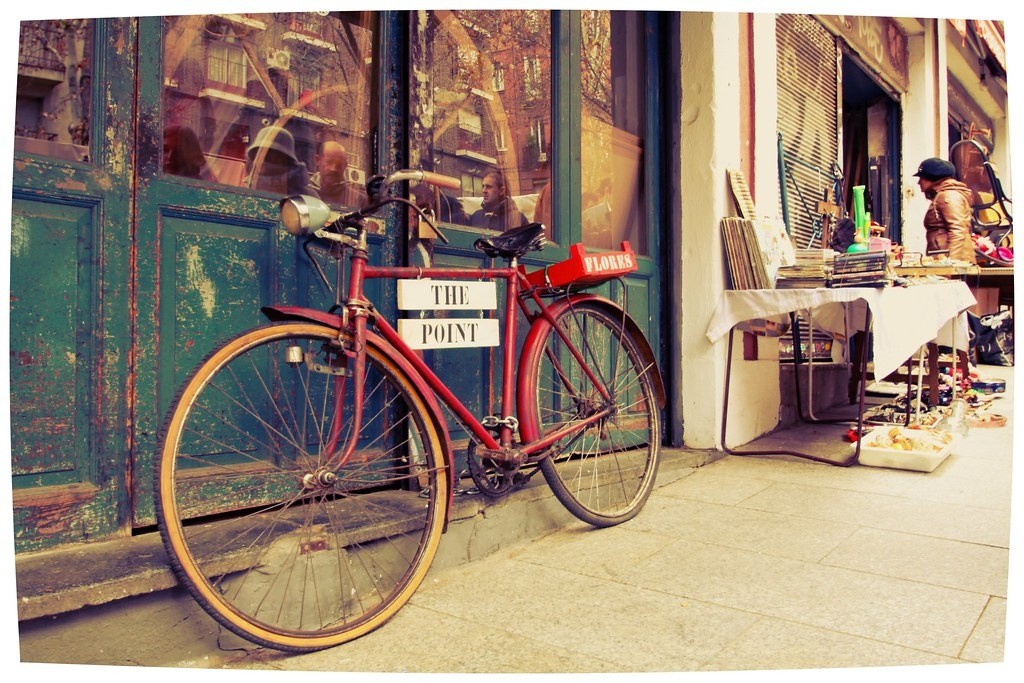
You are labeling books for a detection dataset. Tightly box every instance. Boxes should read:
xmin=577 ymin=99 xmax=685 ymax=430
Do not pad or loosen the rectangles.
xmin=721 ymin=165 xmax=899 ymax=291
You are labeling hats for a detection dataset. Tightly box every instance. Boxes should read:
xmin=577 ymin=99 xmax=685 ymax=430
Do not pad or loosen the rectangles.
xmin=246 ymin=127 xmax=297 ymax=164
xmin=913 ymin=158 xmax=955 ymax=181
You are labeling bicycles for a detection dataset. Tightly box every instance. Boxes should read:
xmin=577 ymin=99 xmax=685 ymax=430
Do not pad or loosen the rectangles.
xmin=153 ymin=167 xmax=668 ymax=655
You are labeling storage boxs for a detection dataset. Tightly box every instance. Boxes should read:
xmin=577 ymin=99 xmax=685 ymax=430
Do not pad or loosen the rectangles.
xmin=852 ymin=428 xmax=958 ymax=473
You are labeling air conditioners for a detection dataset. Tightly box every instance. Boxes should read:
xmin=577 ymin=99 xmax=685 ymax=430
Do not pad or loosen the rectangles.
xmin=260 ymin=47 xmax=290 ymax=71
xmin=345 ymin=167 xmax=366 ymax=186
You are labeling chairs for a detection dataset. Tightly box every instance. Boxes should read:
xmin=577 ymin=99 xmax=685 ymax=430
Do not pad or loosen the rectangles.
xmin=949 ymin=139 xmax=1014 ymax=268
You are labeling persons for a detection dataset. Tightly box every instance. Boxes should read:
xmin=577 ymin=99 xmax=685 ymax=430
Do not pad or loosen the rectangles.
xmin=160 ymin=120 xmax=554 ymax=240
xmin=913 ymin=157 xmax=975 ymax=395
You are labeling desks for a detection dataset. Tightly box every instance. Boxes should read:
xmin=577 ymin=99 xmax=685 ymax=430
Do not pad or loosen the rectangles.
xmin=889 ymin=266 xmax=1014 ymax=319
xmin=705 ymin=280 xmax=977 ymax=467
